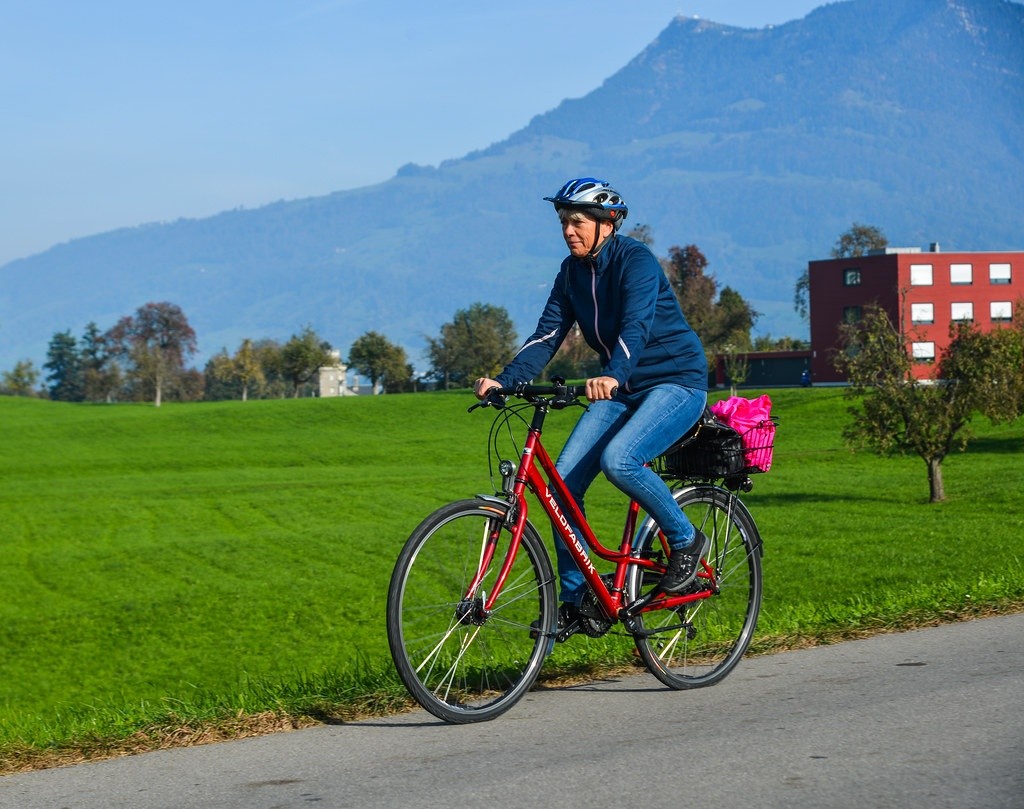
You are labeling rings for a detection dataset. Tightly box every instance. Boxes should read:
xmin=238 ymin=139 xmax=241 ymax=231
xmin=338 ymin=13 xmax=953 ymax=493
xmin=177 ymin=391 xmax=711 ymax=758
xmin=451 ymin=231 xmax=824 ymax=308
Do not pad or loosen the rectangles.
xmin=473 ymin=391 xmax=475 ymax=392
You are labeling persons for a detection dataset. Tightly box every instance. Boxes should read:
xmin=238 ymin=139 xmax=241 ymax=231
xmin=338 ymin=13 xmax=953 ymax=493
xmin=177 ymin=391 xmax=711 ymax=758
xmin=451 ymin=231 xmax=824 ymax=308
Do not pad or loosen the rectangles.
xmin=472 ymin=178 xmax=707 ymax=639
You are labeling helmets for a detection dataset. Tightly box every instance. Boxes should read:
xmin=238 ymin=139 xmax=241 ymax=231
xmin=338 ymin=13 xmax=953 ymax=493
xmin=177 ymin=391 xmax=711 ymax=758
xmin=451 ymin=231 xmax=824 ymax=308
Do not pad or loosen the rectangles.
xmin=542 ymin=177 xmax=628 ymax=219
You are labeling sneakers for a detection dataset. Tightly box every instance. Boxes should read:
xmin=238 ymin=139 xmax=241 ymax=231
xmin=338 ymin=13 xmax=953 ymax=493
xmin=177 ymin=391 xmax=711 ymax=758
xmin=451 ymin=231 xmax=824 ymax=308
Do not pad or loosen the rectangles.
xmin=660 ymin=523 xmax=709 ymax=591
xmin=530 ymin=601 xmax=582 ymax=638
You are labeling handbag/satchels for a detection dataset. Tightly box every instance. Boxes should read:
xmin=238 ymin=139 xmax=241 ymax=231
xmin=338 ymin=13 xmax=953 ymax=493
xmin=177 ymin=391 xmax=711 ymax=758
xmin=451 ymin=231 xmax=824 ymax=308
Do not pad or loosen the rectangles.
xmin=709 ymin=395 xmax=774 ymax=472
xmin=653 ymin=402 xmax=743 ymax=477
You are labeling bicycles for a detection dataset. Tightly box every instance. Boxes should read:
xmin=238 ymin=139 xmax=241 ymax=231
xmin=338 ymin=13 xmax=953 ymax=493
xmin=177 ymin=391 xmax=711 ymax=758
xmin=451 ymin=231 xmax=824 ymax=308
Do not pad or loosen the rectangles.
xmin=386 ymin=374 xmax=781 ymax=724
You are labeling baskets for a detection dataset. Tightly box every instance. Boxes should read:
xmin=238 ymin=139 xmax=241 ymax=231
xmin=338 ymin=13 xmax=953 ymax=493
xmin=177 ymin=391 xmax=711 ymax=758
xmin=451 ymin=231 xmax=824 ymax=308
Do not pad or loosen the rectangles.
xmin=652 ymin=423 xmax=781 ymax=479
